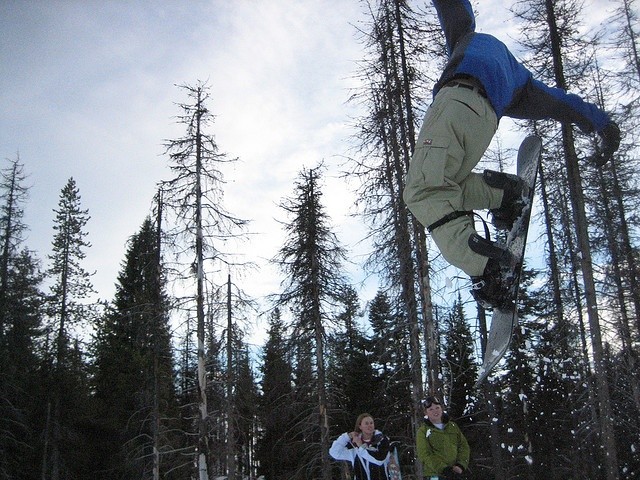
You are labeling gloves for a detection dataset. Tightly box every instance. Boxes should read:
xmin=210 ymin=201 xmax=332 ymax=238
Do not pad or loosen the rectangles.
xmin=442 ymin=466 xmax=456 ymax=478
xmin=594 ymin=120 xmax=620 ymax=167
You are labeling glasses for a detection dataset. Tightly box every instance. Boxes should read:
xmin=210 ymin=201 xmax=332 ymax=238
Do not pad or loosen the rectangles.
xmin=424 ymin=395 xmax=440 ymax=407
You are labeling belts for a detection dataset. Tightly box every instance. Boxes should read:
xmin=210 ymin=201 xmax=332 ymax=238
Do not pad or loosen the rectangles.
xmin=446 ymin=81 xmax=488 ymax=99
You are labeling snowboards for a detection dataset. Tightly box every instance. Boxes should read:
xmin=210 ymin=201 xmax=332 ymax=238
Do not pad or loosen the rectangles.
xmin=384 ymin=440 xmax=403 ymax=480
xmin=471 ymin=135 xmax=545 ymax=390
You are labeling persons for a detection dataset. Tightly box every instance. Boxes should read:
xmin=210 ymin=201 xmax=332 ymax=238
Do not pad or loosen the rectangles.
xmin=329 ymin=413 xmax=390 ymax=479
xmin=416 ymin=395 xmax=471 ymax=480
xmin=402 ymin=0 xmax=621 ymax=310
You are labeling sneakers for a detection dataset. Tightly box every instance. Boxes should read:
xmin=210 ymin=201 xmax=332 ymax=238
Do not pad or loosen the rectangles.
xmin=487 ymin=186 xmax=530 ymax=230
xmin=469 ymin=256 xmax=517 ymax=313
